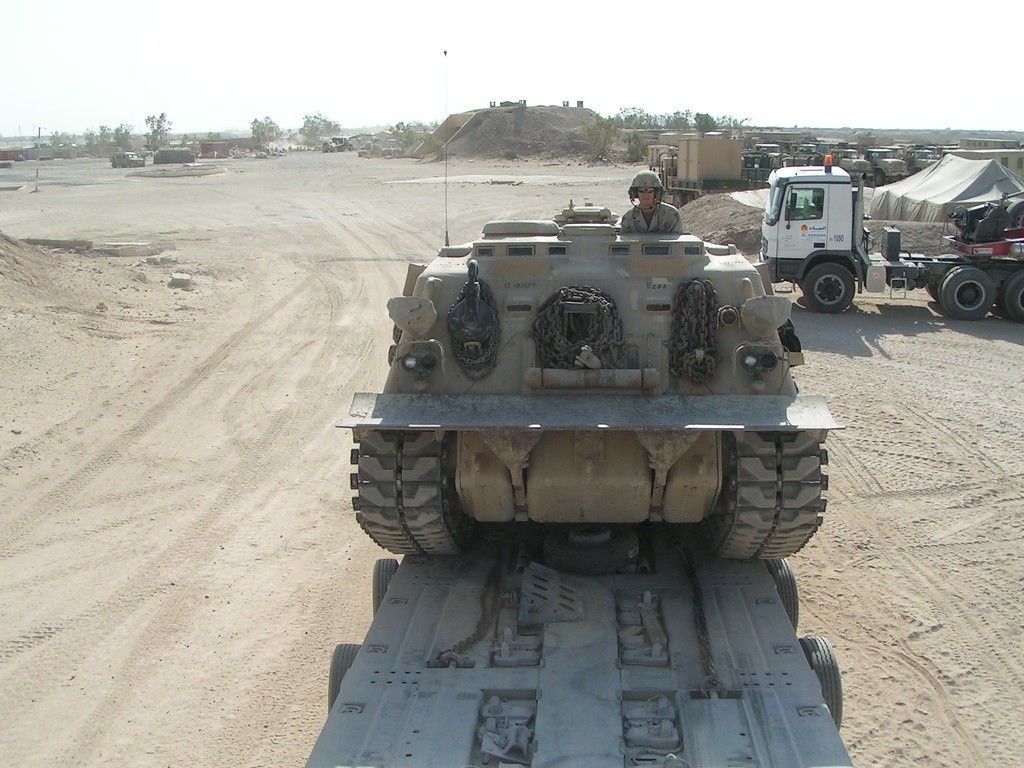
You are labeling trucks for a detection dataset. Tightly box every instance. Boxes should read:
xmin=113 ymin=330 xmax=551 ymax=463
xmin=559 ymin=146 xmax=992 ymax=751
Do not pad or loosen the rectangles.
xmin=647 ymin=130 xmax=949 ymax=204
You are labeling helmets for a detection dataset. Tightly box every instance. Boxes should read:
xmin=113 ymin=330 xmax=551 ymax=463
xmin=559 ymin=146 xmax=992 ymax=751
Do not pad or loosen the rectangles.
xmin=628 ymin=170 xmax=664 ymax=201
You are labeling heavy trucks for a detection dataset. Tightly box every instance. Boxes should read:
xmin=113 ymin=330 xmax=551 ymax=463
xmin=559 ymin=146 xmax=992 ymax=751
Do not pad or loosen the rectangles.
xmin=757 ymin=156 xmax=1024 ymax=324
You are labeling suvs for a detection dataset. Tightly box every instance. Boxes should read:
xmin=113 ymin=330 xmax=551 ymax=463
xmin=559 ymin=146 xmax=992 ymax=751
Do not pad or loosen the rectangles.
xmin=110 ymin=152 xmax=145 ymax=168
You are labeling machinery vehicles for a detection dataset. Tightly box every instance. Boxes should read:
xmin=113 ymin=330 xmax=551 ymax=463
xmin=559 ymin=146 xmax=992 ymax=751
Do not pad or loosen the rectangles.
xmin=342 ymin=199 xmax=848 ymax=575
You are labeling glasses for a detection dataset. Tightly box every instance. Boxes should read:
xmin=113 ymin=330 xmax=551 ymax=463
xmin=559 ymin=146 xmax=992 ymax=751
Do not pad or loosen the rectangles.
xmin=638 ymin=187 xmax=655 ymax=193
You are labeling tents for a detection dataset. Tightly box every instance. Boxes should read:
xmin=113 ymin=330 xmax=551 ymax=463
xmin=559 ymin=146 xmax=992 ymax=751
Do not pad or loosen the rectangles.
xmin=869 ymin=154 xmax=1024 ymax=222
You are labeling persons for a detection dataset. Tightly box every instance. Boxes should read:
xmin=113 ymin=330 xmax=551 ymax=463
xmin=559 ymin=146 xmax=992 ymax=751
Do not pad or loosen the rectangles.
xmin=615 ymin=170 xmax=683 ymax=235
